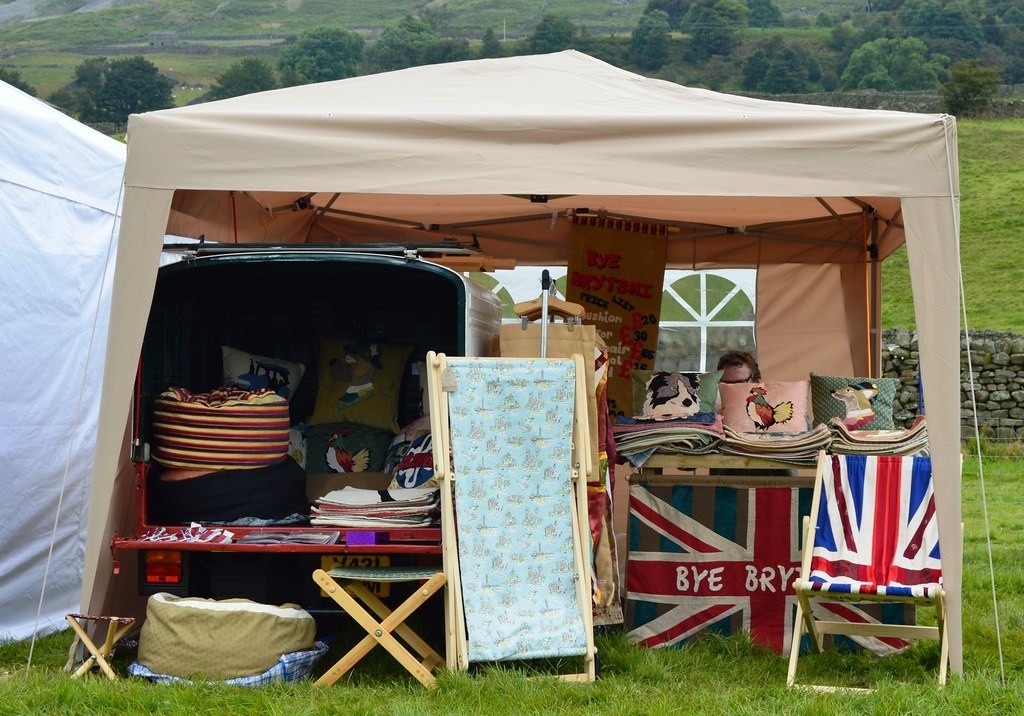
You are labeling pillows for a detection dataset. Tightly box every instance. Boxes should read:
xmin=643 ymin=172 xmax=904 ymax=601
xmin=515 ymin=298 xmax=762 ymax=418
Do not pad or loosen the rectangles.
xmin=303 ymin=423 xmax=391 ymax=474
xmin=809 ymin=370 xmax=900 ymax=433
xmin=306 ymin=336 xmax=416 ymax=432
xmin=386 ymin=428 xmax=440 ymax=488
xmin=718 ymin=382 xmax=810 ymax=433
xmin=628 ymin=369 xmax=725 ymax=418
xmin=218 ymin=346 xmax=309 ymax=401
xmin=382 ymin=413 xmax=431 ymax=473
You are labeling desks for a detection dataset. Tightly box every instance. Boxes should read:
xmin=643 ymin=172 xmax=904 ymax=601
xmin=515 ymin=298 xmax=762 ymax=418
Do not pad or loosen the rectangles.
xmin=609 ymin=449 xmax=824 ymax=642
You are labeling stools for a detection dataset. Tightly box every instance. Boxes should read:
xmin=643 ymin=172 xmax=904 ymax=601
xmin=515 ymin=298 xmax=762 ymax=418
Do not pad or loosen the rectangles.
xmin=62 ymin=611 xmax=138 ymax=684
xmin=310 ymin=564 xmax=449 ymax=694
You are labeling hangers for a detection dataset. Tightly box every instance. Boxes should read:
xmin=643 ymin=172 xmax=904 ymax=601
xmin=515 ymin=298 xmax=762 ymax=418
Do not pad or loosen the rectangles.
xmin=512 ymin=274 xmax=586 ymax=320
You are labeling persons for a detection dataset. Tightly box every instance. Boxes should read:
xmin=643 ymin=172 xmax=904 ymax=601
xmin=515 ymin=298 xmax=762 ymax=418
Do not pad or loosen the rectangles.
xmin=717 ymin=349 xmax=762 ymax=385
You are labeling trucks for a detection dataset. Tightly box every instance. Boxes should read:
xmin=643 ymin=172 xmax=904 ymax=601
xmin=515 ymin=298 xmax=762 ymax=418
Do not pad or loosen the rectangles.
xmin=134 ymin=243 xmax=507 ymax=620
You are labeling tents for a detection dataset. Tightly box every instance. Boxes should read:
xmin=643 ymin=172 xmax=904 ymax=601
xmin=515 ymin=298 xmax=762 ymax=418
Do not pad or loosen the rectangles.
xmin=0 ymin=77 xmax=129 ymax=647
xmin=77 ymin=50 xmax=965 ymax=686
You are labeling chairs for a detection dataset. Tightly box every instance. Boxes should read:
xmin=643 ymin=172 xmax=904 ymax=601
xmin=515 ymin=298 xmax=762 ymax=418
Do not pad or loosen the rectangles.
xmin=784 ymin=448 xmax=966 ymax=696
xmin=425 ymin=351 xmax=600 ymax=685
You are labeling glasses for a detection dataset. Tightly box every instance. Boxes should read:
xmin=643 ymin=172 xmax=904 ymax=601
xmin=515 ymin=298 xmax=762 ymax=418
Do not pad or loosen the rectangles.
xmin=721 ymin=373 xmax=752 ymax=384
